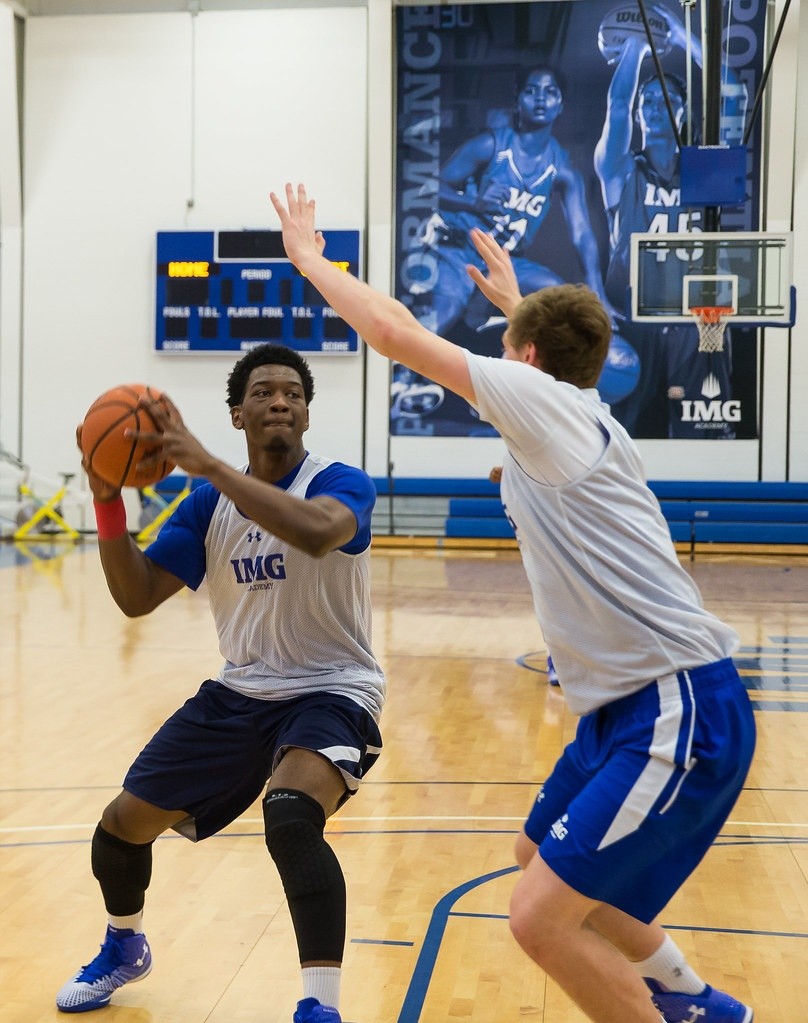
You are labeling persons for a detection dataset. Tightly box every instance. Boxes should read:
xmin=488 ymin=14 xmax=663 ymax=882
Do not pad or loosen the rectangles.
xmin=54 ymin=346 xmax=383 ymax=1020
xmin=267 ymin=182 xmax=759 ymax=1023
xmin=390 ymin=65 xmax=626 ymax=419
xmin=594 ymin=4 xmax=749 ymax=440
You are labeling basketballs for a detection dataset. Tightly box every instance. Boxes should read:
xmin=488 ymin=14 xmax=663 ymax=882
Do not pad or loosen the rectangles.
xmin=597 ymin=2 xmax=666 ymax=68
xmin=595 ymin=335 xmax=639 ymax=405
xmin=82 ymin=385 xmax=181 ymax=487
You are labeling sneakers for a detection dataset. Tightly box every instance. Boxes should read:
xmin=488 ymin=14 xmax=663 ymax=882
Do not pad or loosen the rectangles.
xmin=650 ymin=983 xmax=754 ymax=1023
xmin=56 ymin=923 xmax=152 ymax=1013
xmin=293 ymin=997 xmax=342 ymax=1023
